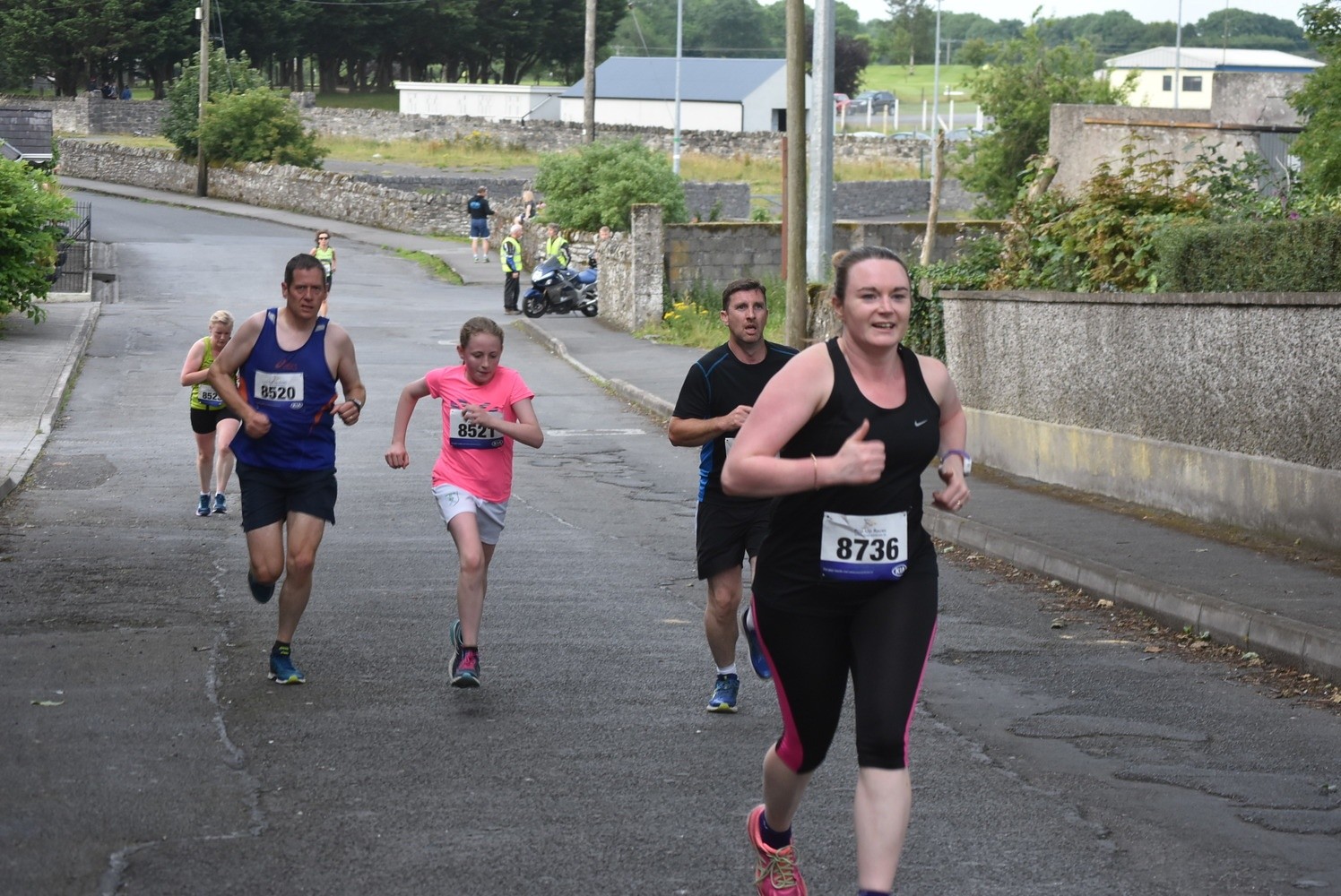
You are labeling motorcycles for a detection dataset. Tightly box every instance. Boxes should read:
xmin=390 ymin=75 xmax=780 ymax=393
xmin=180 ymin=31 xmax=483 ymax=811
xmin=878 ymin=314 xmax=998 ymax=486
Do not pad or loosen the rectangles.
xmin=522 ymin=253 xmax=599 ymax=318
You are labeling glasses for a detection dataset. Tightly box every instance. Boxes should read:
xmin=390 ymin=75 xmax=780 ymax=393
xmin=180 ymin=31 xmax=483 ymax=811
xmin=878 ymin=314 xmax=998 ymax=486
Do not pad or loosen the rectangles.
xmin=319 ymin=237 xmax=328 ymax=240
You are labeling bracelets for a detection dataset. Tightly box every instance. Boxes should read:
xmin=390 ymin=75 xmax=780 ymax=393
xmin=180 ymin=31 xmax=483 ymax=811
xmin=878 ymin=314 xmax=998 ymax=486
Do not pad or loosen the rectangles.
xmin=810 ymin=453 xmax=820 ymax=491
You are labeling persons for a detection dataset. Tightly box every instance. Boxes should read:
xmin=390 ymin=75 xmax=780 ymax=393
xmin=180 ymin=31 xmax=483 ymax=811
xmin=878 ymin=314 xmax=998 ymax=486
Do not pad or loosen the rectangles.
xmin=668 ymin=279 xmax=802 ymax=711
xmin=309 ymin=229 xmax=336 ymax=318
xmin=468 ymin=186 xmax=613 ymax=315
xmin=207 ymin=253 xmax=367 ymax=680
xmin=181 ymin=310 xmax=241 ymax=515
xmin=384 ymin=315 xmax=544 ymax=688
xmin=722 ymin=245 xmax=971 ymax=896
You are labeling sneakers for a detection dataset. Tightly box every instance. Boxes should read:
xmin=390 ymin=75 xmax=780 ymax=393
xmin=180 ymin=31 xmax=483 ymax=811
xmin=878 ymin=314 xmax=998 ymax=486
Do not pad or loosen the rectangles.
xmin=746 ymin=803 xmax=807 ymax=896
xmin=196 ymin=494 xmax=212 ymax=516
xmin=268 ymin=647 xmax=305 ymax=684
xmin=450 ymin=652 xmax=480 ymax=687
xmin=247 ymin=571 xmax=275 ymax=604
xmin=213 ymin=493 xmax=227 ymax=512
xmin=448 ymin=621 xmax=463 ymax=678
xmin=740 ymin=608 xmax=773 ymax=682
xmin=707 ymin=673 xmax=740 ymax=712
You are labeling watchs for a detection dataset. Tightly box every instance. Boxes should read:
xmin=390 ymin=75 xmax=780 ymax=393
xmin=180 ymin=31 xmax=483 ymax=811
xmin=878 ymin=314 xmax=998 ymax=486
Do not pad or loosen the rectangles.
xmin=347 ymin=398 xmax=362 ymax=413
xmin=940 ymin=449 xmax=972 ymax=477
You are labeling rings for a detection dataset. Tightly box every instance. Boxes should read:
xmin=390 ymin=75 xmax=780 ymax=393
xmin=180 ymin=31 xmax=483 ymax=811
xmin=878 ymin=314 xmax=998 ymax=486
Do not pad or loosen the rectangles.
xmin=957 ymin=500 xmax=962 ymax=509
xmin=349 ymin=416 xmax=353 ymax=420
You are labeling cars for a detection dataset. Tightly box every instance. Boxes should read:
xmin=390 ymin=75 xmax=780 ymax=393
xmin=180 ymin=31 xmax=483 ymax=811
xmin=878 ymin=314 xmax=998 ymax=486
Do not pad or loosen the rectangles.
xmin=943 ymin=127 xmax=993 ymax=139
xmin=834 ymin=92 xmax=851 ymax=114
xmin=889 ymin=131 xmax=932 ymax=138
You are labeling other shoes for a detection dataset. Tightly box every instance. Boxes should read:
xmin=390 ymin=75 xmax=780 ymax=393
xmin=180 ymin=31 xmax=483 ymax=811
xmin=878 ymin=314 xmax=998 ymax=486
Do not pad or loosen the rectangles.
xmin=505 ymin=308 xmax=521 ymax=315
xmin=474 ymin=257 xmax=479 ymax=263
xmin=483 ymin=258 xmax=489 ymax=262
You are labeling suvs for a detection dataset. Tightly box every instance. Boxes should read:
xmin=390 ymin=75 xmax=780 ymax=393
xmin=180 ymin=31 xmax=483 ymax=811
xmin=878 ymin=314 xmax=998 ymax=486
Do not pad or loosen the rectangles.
xmin=844 ymin=90 xmax=898 ymax=115
